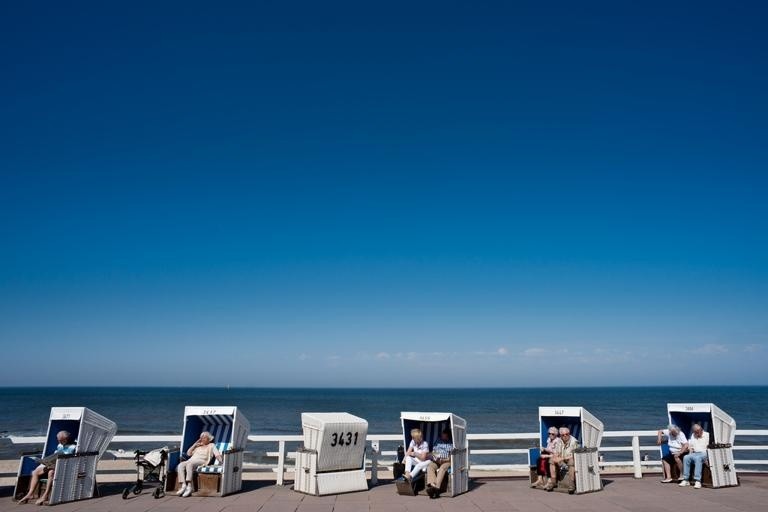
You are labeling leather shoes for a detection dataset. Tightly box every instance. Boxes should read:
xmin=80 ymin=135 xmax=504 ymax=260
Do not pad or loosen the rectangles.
xmin=426 ymin=487 xmax=435 ymax=498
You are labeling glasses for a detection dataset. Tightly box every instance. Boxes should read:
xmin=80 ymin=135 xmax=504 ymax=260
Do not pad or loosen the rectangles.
xmin=548 ymin=433 xmax=555 ymax=436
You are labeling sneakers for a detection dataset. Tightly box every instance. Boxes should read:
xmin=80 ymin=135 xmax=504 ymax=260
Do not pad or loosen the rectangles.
xmin=182 ymin=486 xmax=193 ymax=498
xmin=660 ymin=477 xmax=673 ymax=483
xmin=543 ymin=480 xmax=552 ymax=490
xmin=678 ymin=479 xmax=691 ymax=487
xmin=532 ymin=479 xmax=544 ymax=487
xmin=694 ymin=480 xmax=702 ymax=489
xmin=176 ymin=485 xmax=187 ymax=496
xmin=568 ymin=480 xmax=576 ymax=494
xmin=546 ymin=481 xmax=558 ymax=491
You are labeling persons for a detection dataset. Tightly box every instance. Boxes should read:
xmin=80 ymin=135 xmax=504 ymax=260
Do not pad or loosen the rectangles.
xmin=426 ymin=428 xmax=454 ymax=498
xmin=402 ymin=429 xmax=431 ymax=481
xmin=175 ymin=432 xmax=223 ymax=498
xmin=17 ymin=430 xmax=77 ymax=505
xmin=546 ymin=427 xmax=580 ymax=492
xmin=679 ymin=425 xmax=710 ymax=489
xmin=531 ymin=427 xmax=564 ymax=487
xmin=657 ymin=424 xmax=688 ymax=482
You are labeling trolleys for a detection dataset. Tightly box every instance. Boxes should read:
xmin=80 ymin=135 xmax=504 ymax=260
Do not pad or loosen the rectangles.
xmin=118 ymin=446 xmax=172 ymax=502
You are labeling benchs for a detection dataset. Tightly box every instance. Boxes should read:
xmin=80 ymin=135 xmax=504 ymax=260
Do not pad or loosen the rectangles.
xmin=392 ymin=410 xmax=470 ymax=500
xmin=527 ymin=402 xmax=605 ymax=497
xmin=656 ymin=402 xmax=740 ymax=491
xmin=158 ymin=404 xmax=254 ymax=498
xmin=8 ymin=404 xmax=120 ymax=506
xmin=289 ymin=409 xmax=373 ymax=497
